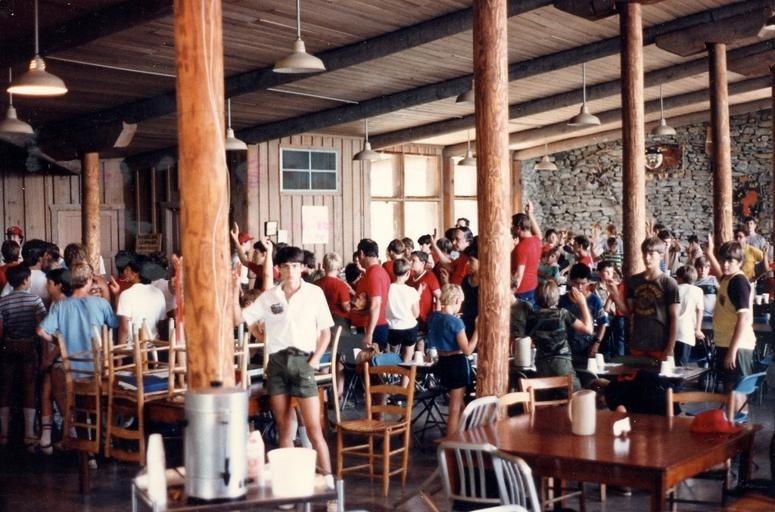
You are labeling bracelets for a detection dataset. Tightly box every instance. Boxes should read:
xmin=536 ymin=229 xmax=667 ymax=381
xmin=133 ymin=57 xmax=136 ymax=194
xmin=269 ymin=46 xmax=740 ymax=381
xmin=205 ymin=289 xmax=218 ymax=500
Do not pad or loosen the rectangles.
xmin=595 ymin=339 xmax=602 ymax=344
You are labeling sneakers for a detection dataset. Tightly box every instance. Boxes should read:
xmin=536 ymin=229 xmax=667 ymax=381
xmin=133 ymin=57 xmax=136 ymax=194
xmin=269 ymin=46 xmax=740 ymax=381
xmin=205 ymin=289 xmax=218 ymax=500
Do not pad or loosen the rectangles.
xmin=27 ymin=440 xmax=53 ymax=455
xmin=122 ymin=415 xmax=134 ymax=428
xmin=607 ymin=485 xmax=632 ymax=496
xmin=278 ymin=503 xmax=295 ymax=510
xmin=88 ymin=459 xmax=98 ymax=469
xmin=0 ymin=438 xmax=8 ymax=445
xmin=326 ymin=500 xmax=338 ymax=512
xmin=24 ymin=433 xmax=40 ymax=445
xmin=54 ymin=436 xmax=80 ymax=451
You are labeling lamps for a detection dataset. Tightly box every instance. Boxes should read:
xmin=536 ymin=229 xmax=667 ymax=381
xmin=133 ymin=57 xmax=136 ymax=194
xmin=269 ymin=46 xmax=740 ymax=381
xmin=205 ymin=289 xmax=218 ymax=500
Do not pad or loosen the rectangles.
xmin=223 ymin=97 xmax=247 ymax=151
xmin=5 ymin=0 xmax=70 ymax=99
xmin=652 ymin=82 xmax=676 ymax=140
xmin=564 ymin=58 xmax=602 ymax=129
xmin=1 ymin=67 xmax=37 ymax=135
xmin=274 ymin=1 xmax=324 ymax=76
xmin=535 ymin=140 xmax=558 ymax=172
xmin=454 ymin=74 xmax=477 ymax=104
xmin=351 ymin=118 xmax=381 ymax=164
xmin=458 ymin=126 xmax=478 ymax=167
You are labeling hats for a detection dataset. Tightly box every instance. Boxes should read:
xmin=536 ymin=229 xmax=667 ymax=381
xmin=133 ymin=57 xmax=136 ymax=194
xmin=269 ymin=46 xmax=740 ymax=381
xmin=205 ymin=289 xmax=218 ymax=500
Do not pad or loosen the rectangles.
xmin=302 ymin=251 xmax=315 ymax=265
xmin=401 ymin=238 xmax=414 ymax=248
xmin=5 ymin=226 xmax=24 ymax=237
xmin=239 ymin=232 xmax=254 ymax=243
xmin=689 ymin=408 xmax=744 ymax=433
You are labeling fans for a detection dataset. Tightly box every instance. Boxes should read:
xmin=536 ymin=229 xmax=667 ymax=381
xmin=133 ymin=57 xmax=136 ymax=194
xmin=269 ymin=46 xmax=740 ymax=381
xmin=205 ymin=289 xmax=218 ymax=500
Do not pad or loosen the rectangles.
xmin=759 ymin=7 xmax=775 ymax=38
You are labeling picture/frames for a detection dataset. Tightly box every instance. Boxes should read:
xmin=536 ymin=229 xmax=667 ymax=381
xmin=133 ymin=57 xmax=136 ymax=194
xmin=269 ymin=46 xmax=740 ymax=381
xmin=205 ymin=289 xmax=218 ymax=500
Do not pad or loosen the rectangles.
xmin=645 ymin=144 xmax=683 ymax=171
xmin=264 ymin=221 xmax=278 ymax=236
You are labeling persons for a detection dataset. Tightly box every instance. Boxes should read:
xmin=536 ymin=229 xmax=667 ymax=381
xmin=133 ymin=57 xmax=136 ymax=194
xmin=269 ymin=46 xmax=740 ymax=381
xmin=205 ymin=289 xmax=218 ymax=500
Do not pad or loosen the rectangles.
xmin=1 ymin=239 xmax=51 ymax=307
xmin=606 ymin=236 xmax=680 ymax=380
xmin=646 ymin=220 xmax=681 ymax=278
xmin=44 ymin=242 xmax=68 ymax=271
xmin=402 ymin=237 xmax=414 ymax=263
xmin=231 ymin=246 xmax=338 ymax=512
xmin=0 ymin=264 xmax=46 ymax=445
xmin=431 ymin=238 xmax=454 ymax=285
xmin=456 ymin=217 xmax=470 ymax=227
xmin=234 ymin=289 xmax=265 ymax=369
xmin=604 ymin=263 xmax=624 ymax=358
xmin=107 ymin=249 xmax=138 ymax=293
xmin=671 ymin=265 xmax=706 ymax=368
xmin=27 ymin=268 xmax=79 ymax=455
xmin=426 ymin=283 xmax=479 ymax=438
xmin=231 ymin=232 xmax=254 ymax=289
xmin=115 ymin=258 xmax=167 ymax=371
xmin=349 ymin=238 xmax=391 ymax=423
xmin=301 ymin=250 xmax=324 ymax=284
xmin=0 ymin=225 xmax=24 ymax=265
xmin=742 ymin=216 xmax=769 ymax=250
xmin=445 ymin=227 xmax=458 ymax=243
xmin=429 ymin=226 xmax=473 ymax=285
xmin=703 ymin=233 xmax=756 ymax=424
xmin=584 ymin=366 xmax=686 ymax=497
xmin=385 ymin=258 xmax=423 ymax=407
xmin=509 ymin=275 xmax=535 ymax=359
xmin=594 ymin=260 xmax=615 ymax=362
xmin=762 ymin=232 xmax=774 ymax=299
xmin=382 ymin=239 xmax=405 ymax=284
xmin=537 ymin=221 xmax=623 ymax=283
xmin=658 ymin=230 xmax=671 ymax=276
xmin=558 ymin=263 xmax=610 ymax=371
xmin=230 ymin=222 xmax=281 ymax=292
xmin=345 ymin=251 xmax=367 ymax=290
xmin=260 ymin=235 xmax=312 ymax=449
xmin=693 ymin=256 xmax=720 ymax=346
xmin=524 ymin=279 xmax=594 ymax=398
xmin=684 ymin=234 xmax=703 ymax=266
xmin=36 ymin=262 xmax=121 ymax=469
xmin=440 ymin=243 xmax=479 ymax=343
xmin=64 ymin=242 xmax=111 ymax=301
xmin=732 ymin=229 xmax=767 ymax=297
xmin=417 ymin=234 xmax=435 ymax=269
xmin=0 ymin=240 xmax=21 ymax=291
xmin=510 ymin=200 xmax=542 ymax=311
xmin=404 ymin=251 xmax=441 ymax=362
xmin=313 ymin=251 xmax=356 ymax=409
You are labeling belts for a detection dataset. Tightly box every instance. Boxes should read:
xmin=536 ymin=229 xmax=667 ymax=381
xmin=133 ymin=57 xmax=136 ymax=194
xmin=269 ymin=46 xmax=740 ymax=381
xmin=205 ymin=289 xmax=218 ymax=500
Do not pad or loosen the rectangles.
xmin=281 ymin=348 xmax=313 ymax=356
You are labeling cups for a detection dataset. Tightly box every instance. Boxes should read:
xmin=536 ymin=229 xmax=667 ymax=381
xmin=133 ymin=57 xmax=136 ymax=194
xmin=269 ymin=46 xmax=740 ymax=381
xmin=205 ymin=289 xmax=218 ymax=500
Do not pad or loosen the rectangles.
xmin=428 ymin=346 xmax=438 ymax=360
xmin=761 ymin=291 xmax=771 ymax=305
xmin=265 ymin=447 xmax=317 ymax=498
xmin=754 ymin=294 xmax=763 ymax=305
xmin=568 ymin=389 xmax=596 ymax=437
xmin=660 ymin=359 xmax=673 ymax=379
xmin=135 ymin=466 xmax=187 ymax=491
xmin=665 ymin=357 xmax=676 ymax=371
xmin=515 ymin=334 xmax=532 ymax=370
xmin=594 ymin=352 xmax=605 ymax=371
xmin=414 ymin=350 xmax=424 ymax=365
xmin=146 ymin=433 xmax=168 ymax=505
xmin=246 ymin=431 xmax=266 ymax=487
xmin=587 ymin=358 xmax=599 ymax=375
xmin=472 ymin=353 xmax=478 ymax=366
